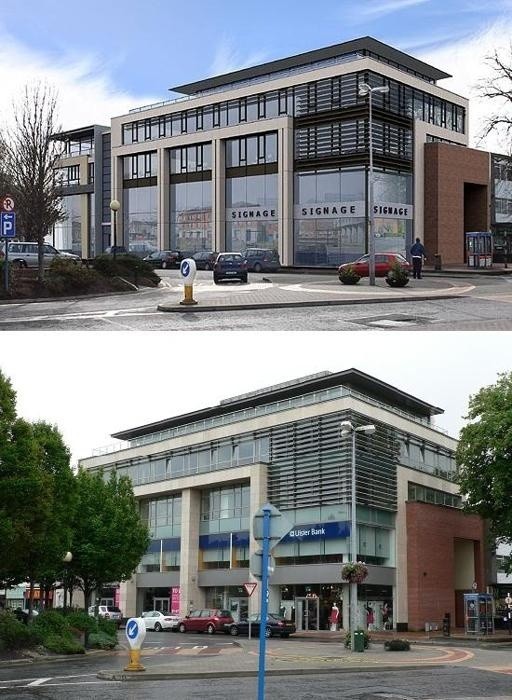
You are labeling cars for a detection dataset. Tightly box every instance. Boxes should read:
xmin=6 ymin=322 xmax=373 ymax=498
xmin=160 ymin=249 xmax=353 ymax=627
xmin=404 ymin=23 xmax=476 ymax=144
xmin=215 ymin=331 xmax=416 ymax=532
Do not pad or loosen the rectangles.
xmin=143 ymin=248 xmax=184 ymax=269
xmin=104 ymin=246 xmax=126 ymax=256
xmin=338 ymin=251 xmax=414 ymax=277
xmin=224 ymin=613 xmax=296 ymax=641
xmin=140 ymin=609 xmax=181 ymax=632
xmin=189 ymin=250 xmax=218 ymax=271
xmin=479 ymin=609 xmax=511 ymax=633
xmin=13 ymin=607 xmax=41 ymax=623
xmin=212 ymin=251 xmax=248 ymax=286
xmin=298 ymin=245 xmax=327 ymax=264
xmin=238 ymin=247 xmax=281 ymax=273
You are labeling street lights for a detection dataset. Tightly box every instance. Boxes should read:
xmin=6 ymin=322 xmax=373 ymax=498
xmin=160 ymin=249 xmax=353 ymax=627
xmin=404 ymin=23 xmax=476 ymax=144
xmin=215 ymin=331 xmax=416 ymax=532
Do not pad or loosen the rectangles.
xmin=62 ymin=551 xmax=73 ymax=619
xmin=357 ymin=79 xmax=390 ymax=283
xmin=336 ymin=418 xmax=378 ymax=652
xmin=109 ymin=200 xmax=122 ymax=261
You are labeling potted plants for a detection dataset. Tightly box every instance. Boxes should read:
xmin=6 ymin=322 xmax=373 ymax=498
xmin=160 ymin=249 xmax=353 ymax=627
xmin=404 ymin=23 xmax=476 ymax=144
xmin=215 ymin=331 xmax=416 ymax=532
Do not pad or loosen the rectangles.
xmin=385 ymin=257 xmax=409 ymax=288
xmin=339 ymin=266 xmax=361 ymax=284
xmin=341 ymin=560 xmax=368 ymax=584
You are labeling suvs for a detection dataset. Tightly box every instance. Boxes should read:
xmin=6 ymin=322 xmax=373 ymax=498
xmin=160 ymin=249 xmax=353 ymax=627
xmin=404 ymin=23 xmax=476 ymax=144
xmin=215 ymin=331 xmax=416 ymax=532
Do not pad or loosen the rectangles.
xmin=178 ymin=608 xmax=235 ymax=634
xmin=0 ymin=241 xmax=82 ymax=271
xmin=86 ymin=606 xmax=123 ymax=629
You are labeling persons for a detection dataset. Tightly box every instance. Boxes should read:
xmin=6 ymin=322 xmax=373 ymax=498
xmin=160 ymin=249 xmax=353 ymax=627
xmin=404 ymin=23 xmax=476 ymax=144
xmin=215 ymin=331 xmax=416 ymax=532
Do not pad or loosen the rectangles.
xmin=409 ymin=238 xmax=428 ymax=279
xmin=330 ymin=602 xmax=339 ymax=631
xmin=380 ymin=603 xmax=388 ymax=620
